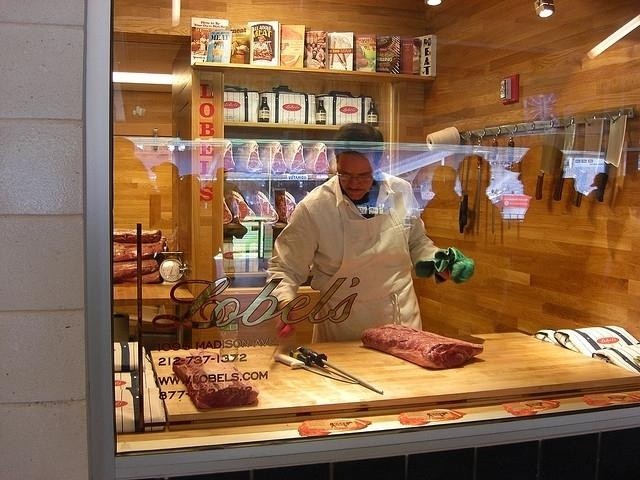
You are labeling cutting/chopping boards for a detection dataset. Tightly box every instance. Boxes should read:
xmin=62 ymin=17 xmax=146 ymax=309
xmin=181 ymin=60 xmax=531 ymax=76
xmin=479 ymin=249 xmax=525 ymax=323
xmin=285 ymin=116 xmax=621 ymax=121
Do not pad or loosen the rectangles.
xmin=150 ymin=331 xmax=640 ymax=433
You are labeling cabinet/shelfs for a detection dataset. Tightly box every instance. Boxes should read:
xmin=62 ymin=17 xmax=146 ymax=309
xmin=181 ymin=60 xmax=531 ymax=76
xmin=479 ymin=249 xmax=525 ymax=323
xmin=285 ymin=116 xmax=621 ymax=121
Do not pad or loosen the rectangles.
xmin=171 ymin=42 xmax=436 ymax=347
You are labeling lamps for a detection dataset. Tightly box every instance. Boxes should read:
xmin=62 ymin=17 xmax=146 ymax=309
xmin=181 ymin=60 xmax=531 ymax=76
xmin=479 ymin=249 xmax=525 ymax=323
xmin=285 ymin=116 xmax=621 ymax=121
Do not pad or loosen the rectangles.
xmin=112 ymin=72 xmax=173 ymax=84
xmin=425 ymin=0 xmax=443 ymax=7
xmin=587 ymin=14 xmax=640 ymax=60
xmin=535 ymin=0 xmax=555 ymax=19
xmin=171 ymin=0 xmax=181 ymax=27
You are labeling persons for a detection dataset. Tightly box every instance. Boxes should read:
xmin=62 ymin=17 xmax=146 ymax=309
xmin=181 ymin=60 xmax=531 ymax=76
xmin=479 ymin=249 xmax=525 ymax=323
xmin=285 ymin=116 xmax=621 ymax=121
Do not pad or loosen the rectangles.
xmin=265 ymin=122 xmax=475 ymax=344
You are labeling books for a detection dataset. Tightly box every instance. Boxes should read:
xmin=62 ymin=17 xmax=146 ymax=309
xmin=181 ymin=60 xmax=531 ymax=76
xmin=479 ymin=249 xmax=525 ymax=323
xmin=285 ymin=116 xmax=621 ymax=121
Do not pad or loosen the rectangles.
xmin=190 ymin=15 xmax=436 ymax=77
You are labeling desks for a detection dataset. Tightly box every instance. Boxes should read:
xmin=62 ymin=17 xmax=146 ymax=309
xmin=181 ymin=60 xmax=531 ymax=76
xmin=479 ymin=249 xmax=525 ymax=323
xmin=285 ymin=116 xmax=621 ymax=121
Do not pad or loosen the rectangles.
xmin=111 ymin=283 xmax=195 ymax=349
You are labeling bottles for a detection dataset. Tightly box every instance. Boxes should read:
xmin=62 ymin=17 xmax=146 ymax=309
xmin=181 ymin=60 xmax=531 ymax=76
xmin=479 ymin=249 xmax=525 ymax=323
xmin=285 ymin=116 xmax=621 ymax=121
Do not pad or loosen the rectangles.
xmin=259 ymin=97 xmax=269 ymax=123
xmin=315 ymin=100 xmax=327 ymax=124
xmin=367 ymin=102 xmax=379 ymax=128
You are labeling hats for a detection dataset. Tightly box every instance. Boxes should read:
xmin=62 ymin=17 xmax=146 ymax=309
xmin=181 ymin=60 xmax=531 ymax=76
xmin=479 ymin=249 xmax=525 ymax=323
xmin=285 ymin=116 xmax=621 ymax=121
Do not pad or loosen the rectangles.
xmin=589 ymin=171 xmax=609 ymax=188
xmin=503 ymin=143 xmax=564 ymax=174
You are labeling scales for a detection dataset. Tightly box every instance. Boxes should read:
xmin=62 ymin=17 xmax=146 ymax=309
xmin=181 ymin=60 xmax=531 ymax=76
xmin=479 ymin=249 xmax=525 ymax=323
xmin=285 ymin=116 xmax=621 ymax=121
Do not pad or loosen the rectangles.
xmin=159 ymin=252 xmax=188 ymax=285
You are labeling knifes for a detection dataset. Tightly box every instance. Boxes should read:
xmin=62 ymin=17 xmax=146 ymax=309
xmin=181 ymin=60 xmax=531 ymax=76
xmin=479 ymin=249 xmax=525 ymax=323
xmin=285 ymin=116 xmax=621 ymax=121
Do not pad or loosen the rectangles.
xmin=534 ymin=116 xmax=628 ymax=207
